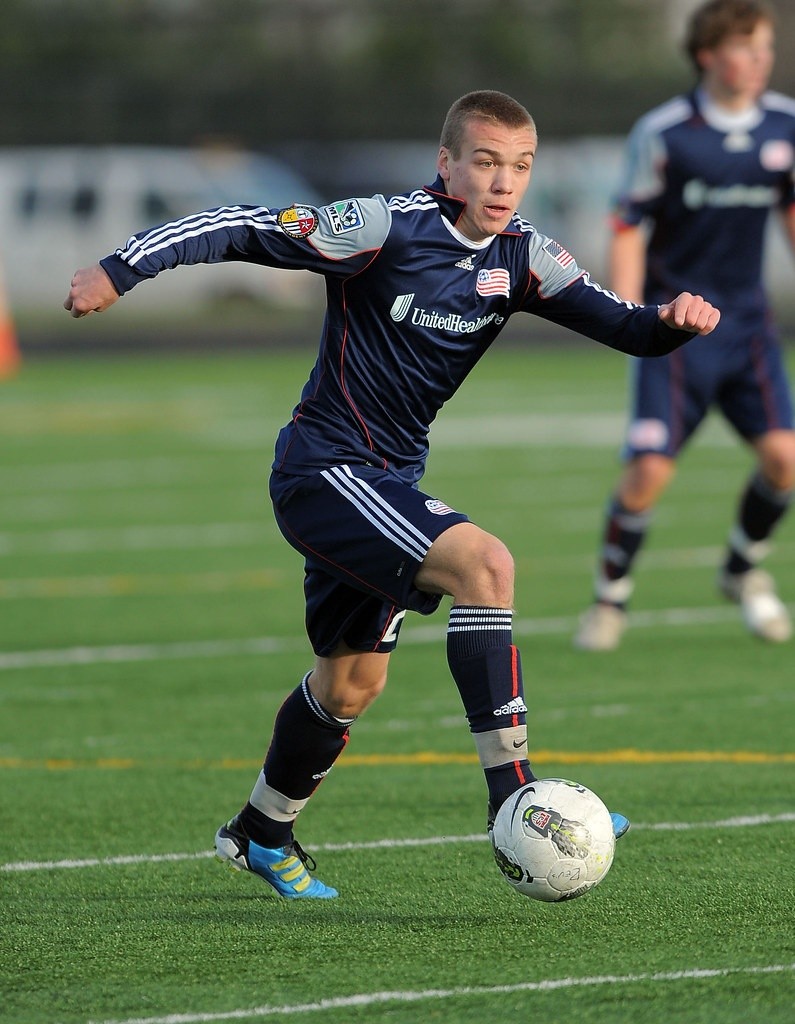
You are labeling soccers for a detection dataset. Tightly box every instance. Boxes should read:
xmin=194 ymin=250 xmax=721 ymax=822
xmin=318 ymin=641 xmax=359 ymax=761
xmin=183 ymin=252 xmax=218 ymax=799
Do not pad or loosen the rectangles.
xmin=492 ymin=777 xmax=618 ymax=903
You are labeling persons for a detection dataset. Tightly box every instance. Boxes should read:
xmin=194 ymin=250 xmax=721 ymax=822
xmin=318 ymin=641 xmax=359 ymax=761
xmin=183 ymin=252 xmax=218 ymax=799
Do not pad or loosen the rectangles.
xmin=572 ymin=0 xmax=795 ymax=652
xmin=65 ymin=90 xmax=721 ymax=901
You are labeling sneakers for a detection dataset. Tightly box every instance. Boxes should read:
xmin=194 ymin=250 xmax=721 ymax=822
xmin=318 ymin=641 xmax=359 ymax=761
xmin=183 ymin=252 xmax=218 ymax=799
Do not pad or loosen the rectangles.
xmin=487 ymin=799 xmax=629 ymax=846
xmin=214 ymin=802 xmax=339 ymax=900
xmin=716 ymin=565 xmax=792 ymax=642
xmin=572 ymin=602 xmax=627 ymax=649
